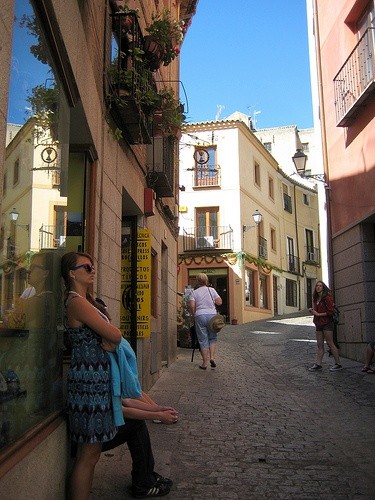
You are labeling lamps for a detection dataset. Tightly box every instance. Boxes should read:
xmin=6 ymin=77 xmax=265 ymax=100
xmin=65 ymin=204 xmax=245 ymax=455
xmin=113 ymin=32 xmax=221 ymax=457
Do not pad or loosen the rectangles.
xmin=243 ymin=209 xmax=262 ymax=238
xmin=292 ymin=149 xmax=328 ymax=186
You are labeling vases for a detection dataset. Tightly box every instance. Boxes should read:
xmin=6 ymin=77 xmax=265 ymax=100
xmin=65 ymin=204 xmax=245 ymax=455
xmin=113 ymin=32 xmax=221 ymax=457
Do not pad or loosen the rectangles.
xmin=144 ymin=35 xmax=164 ymax=70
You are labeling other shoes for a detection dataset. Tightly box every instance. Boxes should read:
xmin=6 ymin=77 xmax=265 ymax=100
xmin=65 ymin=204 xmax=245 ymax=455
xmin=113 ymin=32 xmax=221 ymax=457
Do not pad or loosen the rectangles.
xmin=366 ymin=368 xmax=375 ymax=373
xmin=311 ymin=364 xmax=322 ymax=369
xmin=361 ymin=365 xmax=370 ymax=372
xmin=331 ymin=363 xmax=342 ymax=369
xmin=199 ymin=365 xmax=207 ymax=369
xmin=210 ymin=360 xmax=216 ymax=367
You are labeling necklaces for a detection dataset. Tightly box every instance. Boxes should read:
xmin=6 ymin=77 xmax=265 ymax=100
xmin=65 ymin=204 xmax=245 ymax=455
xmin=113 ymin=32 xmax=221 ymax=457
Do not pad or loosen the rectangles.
xmin=69 ymin=291 xmax=109 ymax=323
xmin=38 ymin=290 xmax=54 ymax=296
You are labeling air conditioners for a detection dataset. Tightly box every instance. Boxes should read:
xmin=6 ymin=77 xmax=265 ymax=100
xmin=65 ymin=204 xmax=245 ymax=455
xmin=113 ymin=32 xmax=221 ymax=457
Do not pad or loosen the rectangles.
xmin=308 ymin=253 xmax=315 ymax=260
xmin=195 ymin=235 xmax=214 ymax=249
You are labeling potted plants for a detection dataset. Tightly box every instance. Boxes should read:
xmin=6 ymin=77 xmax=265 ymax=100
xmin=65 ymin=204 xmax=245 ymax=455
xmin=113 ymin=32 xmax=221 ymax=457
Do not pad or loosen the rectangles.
xmin=108 ymin=0 xmax=188 ymax=144
xmin=179 ymin=293 xmax=194 ymax=347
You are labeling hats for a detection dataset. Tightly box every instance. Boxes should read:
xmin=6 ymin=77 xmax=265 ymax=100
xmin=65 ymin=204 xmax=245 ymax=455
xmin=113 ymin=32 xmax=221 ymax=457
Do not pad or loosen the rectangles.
xmin=209 ymin=315 xmax=225 ymax=333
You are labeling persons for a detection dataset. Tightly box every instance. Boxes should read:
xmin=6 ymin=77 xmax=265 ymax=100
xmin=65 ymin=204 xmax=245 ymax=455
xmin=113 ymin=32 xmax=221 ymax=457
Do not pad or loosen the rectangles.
xmin=189 ymin=273 xmax=222 ymax=369
xmin=7 ymin=250 xmax=57 ymax=421
xmin=59 ymin=252 xmax=122 ymax=500
xmin=308 ymin=280 xmax=343 ymax=372
xmin=361 ymin=339 xmax=375 ymax=373
xmin=95 ymin=298 xmax=179 ymax=497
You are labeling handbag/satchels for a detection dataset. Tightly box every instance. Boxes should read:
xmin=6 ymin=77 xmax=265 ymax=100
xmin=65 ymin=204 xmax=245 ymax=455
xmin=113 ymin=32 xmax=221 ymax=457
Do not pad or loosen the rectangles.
xmin=332 ymin=308 xmax=340 ymax=324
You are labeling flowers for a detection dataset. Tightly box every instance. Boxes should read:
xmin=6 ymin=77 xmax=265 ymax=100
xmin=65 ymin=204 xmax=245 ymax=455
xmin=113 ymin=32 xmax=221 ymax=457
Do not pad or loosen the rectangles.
xmin=145 ymin=7 xmax=191 ymax=65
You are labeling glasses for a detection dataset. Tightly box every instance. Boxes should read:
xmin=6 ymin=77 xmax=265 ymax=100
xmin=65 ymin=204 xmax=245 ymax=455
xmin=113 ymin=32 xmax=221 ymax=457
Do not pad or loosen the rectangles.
xmin=29 ymin=263 xmax=44 ymax=271
xmin=72 ymin=264 xmax=96 ymax=272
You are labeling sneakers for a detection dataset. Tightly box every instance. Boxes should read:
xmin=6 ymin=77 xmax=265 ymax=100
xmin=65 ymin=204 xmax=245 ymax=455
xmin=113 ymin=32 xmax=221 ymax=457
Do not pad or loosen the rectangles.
xmin=130 ymin=471 xmax=174 ymax=499
